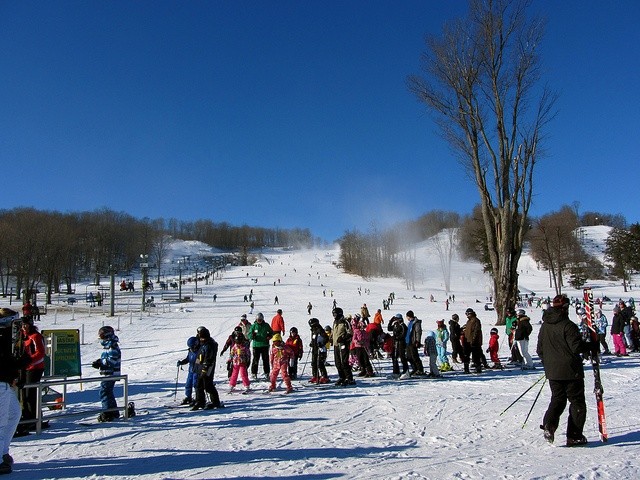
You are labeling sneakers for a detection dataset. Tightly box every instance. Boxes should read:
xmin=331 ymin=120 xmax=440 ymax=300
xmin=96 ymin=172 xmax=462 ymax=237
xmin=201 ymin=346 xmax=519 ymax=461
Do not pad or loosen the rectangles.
xmin=192 ymin=403 xmax=206 ymax=409
xmin=320 ymin=376 xmax=330 ymax=382
xmin=269 ymin=385 xmax=275 ymax=390
xmin=544 ymin=424 xmax=554 ymax=443
xmin=0 ymin=463 xmax=11 ymax=475
xmin=440 ymin=363 xmax=451 ymax=370
xmin=566 ymin=434 xmax=587 ymax=446
xmin=344 ymin=378 xmax=356 ymax=385
xmin=245 ymin=385 xmax=250 ymax=391
xmin=229 ymin=386 xmax=234 ymax=392
xmin=184 ymin=397 xmax=191 ymax=402
xmin=310 ymin=377 xmax=319 ymax=381
xmin=211 ymin=401 xmax=221 ymax=408
xmin=286 ymin=388 xmax=293 ymax=391
xmin=401 ymin=373 xmax=409 ymax=379
xmin=4 ymin=453 xmax=13 ymax=463
xmin=335 ymin=379 xmax=346 ymax=383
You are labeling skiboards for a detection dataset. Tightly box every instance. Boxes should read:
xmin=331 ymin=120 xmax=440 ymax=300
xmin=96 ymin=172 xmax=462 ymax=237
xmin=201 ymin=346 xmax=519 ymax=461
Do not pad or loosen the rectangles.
xmin=583 ymin=288 xmax=608 ymax=444
xmin=164 ymin=399 xmax=211 ymax=408
xmin=314 ymin=380 xmax=370 ymax=390
xmin=263 ymin=384 xmax=312 ymax=397
xmin=227 ymin=387 xmax=266 ymax=395
xmin=167 ymin=402 xmax=237 ymax=420
xmin=299 ymin=379 xmax=337 ymax=388
xmin=75 ymin=409 xmax=149 ymax=427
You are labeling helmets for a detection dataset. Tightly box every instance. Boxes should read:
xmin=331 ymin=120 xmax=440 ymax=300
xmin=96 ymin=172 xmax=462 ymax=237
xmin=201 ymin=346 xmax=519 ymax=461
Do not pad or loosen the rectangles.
xmin=97 ymin=327 xmax=114 ymax=340
xmin=491 ymin=328 xmax=498 ymax=334
xmin=507 ymin=307 xmax=514 ymax=313
xmin=309 ymin=319 xmax=318 ymax=327
xmin=187 ymin=337 xmax=199 ymax=347
xmin=332 ymin=308 xmax=342 ymax=316
xmin=517 ymin=310 xmax=525 ymax=315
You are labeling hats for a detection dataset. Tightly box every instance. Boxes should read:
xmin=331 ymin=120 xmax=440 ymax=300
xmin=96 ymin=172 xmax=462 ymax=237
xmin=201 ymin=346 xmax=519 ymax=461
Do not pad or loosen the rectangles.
xmin=614 ymin=307 xmax=621 ymax=313
xmin=256 ymin=313 xmax=263 ymax=320
xmin=396 ymin=314 xmax=402 ymax=319
xmin=235 ymin=326 xmax=242 ymax=332
xmin=273 ymin=333 xmax=281 ymax=340
xmin=465 ymin=308 xmax=473 ymax=313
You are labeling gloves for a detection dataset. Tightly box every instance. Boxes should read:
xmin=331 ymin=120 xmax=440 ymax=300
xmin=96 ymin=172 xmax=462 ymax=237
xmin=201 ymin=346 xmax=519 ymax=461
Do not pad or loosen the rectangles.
xmin=96 ymin=359 xmax=102 ymax=367
xmin=177 ymin=361 xmax=181 ymax=366
xmin=92 ymin=361 xmax=97 ymax=368
xmin=199 ymin=371 xmax=206 ymax=379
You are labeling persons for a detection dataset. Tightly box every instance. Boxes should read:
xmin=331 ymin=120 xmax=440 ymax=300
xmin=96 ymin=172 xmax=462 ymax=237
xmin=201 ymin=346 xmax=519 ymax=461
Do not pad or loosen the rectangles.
xmin=119 ymin=280 xmax=134 ymax=292
xmin=611 ymin=298 xmax=640 ymax=357
xmin=576 ymin=307 xmax=609 ymax=363
xmin=516 ymin=290 xmax=536 ymax=306
xmin=504 ymin=307 xmax=534 ymax=369
xmin=177 ymin=326 xmax=221 ymax=411
xmin=0 ymin=381 xmax=23 ymax=475
xmin=220 ymin=289 xmax=303 ymax=394
xmin=424 ymin=294 xmax=503 ymax=378
xmin=92 ymin=326 xmax=121 ymax=422
xmin=21 ymin=300 xmax=40 ymax=322
xmin=536 ymin=294 xmax=611 ymax=447
xmin=14 ymin=317 xmax=47 ymax=437
xmin=307 ymin=292 xmax=424 ymax=386
